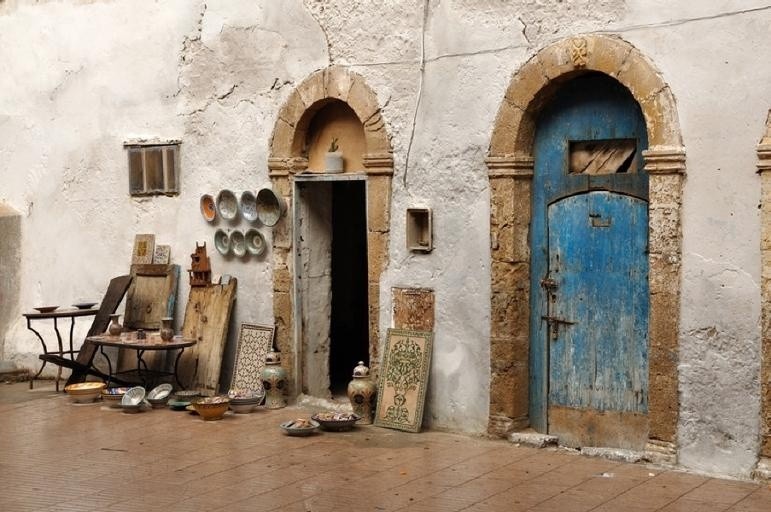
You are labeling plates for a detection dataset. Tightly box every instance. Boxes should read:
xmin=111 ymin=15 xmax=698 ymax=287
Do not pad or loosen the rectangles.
xmin=71 ymin=302 xmax=97 ymax=310
xmin=311 ymin=414 xmax=361 ymax=431
xmin=279 ymin=420 xmax=320 ymax=436
xmin=32 ymin=305 xmax=60 ymax=314
xmin=170 ymin=401 xmax=195 ymax=412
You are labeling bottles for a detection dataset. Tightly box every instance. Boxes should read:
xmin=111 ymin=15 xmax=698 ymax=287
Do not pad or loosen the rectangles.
xmin=159 ymin=318 xmax=175 ymax=343
xmin=109 ymin=314 xmax=123 ymax=339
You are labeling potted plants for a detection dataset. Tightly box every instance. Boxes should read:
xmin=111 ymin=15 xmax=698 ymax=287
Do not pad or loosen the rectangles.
xmin=325 ymin=137 xmax=344 ymax=174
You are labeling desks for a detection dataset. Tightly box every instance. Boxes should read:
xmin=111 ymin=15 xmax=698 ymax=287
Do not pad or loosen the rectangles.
xmin=85 ymin=329 xmax=198 ymax=393
xmin=22 ymin=309 xmax=112 ymax=393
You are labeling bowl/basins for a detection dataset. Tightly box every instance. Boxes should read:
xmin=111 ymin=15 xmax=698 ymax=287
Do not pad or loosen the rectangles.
xmin=257 ymin=188 xmax=287 ymax=227
xmin=200 ymin=194 xmax=216 ymax=222
xmin=244 ymin=227 xmax=266 ymax=255
xmin=174 ymin=391 xmax=201 ymax=400
xmin=229 ymin=230 xmax=247 ymax=258
xmin=146 ymin=383 xmax=173 ymax=409
xmin=103 ymin=386 xmax=146 ymax=414
xmin=214 ymin=228 xmax=230 ymax=256
xmin=238 ymin=191 xmax=257 ymax=222
xmin=190 ymin=396 xmax=232 ymax=421
xmin=228 ymin=396 xmax=260 ymax=414
xmin=65 ymin=382 xmax=106 ymax=404
xmin=216 ymin=190 xmax=237 ymax=221
